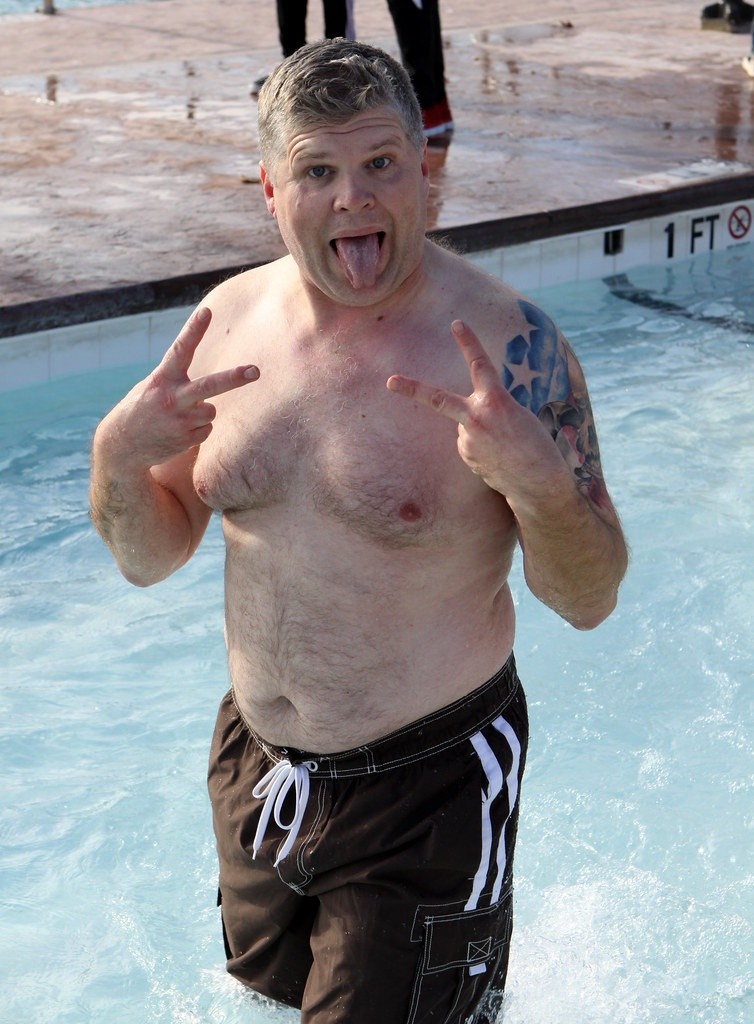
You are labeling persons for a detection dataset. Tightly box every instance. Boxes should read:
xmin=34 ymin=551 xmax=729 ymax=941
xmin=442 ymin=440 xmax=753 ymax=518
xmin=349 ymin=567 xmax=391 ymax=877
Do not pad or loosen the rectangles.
xmin=250 ymin=1 xmax=456 ymax=141
xmin=87 ymin=38 xmax=631 ymax=1023
